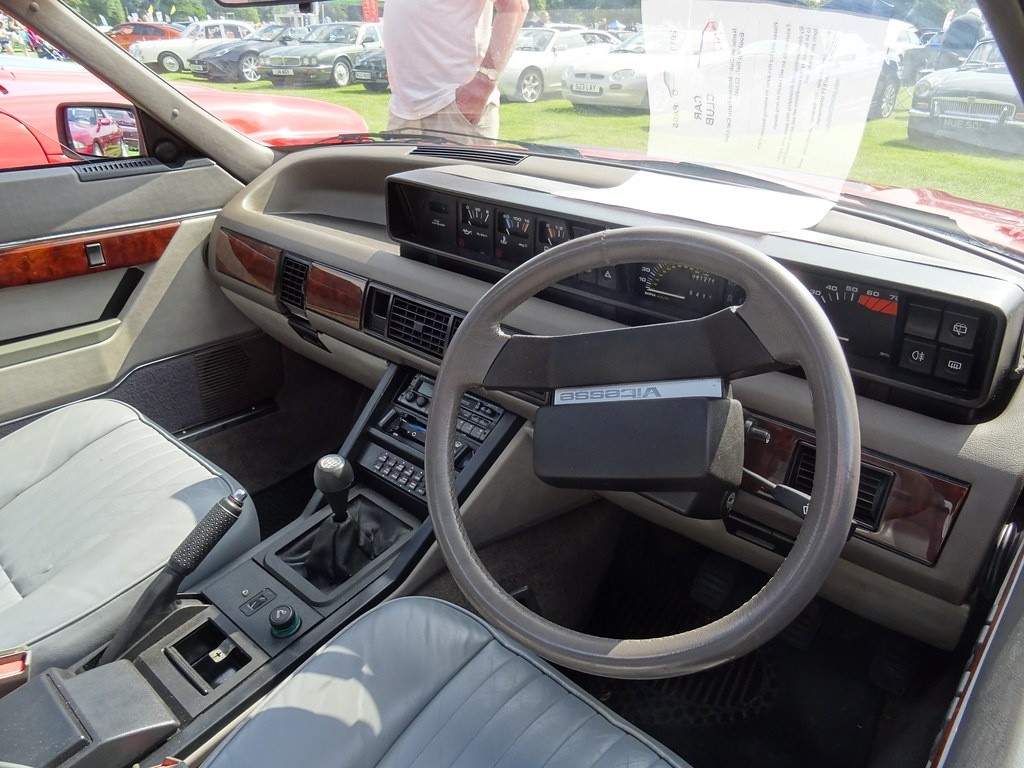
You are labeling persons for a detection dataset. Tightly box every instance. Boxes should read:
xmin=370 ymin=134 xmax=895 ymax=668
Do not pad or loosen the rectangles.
xmin=533 ymin=10 xmax=549 ymax=28
xmin=598 ymin=17 xmax=637 ymax=33
xmin=383 ymin=0 xmax=527 ymax=146
xmin=0 ymin=11 xmax=65 ymax=60
xmin=932 ymin=8 xmax=984 ymax=71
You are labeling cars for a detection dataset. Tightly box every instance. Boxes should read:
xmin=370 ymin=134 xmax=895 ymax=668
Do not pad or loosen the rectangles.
xmin=496 ymin=26 xmax=585 ymax=103
xmin=352 ymin=46 xmax=391 ymax=93
xmin=0 ymin=46 xmax=371 ymax=169
xmin=522 ymin=21 xmax=646 ymax=56
xmin=64 ymin=105 xmax=129 ymax=159
xmin=907 ymin=35 xmax=1024 ymax=162
xmin=897 ymin=27 xmax=977 ymax=88
xmin=865 ymin=54 xmax=900 ymax=121
xmin=102 ymin=20 xmax=189 ymax=56
xmin=187 ymin=23 xmax=308 ymax=85
xmin=259 ymin=19 xmax=387 ymax=89
xmin=127 ymin=19 xmax=258 ymax=73
xmin=560 ymin=29 xmax=650 ymax=114
xmin=104 ymin=109 xmax=139 ymax=148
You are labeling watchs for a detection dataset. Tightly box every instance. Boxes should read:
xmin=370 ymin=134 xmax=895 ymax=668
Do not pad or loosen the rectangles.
xmin=478 ymin=66 xmax=500 ymax=83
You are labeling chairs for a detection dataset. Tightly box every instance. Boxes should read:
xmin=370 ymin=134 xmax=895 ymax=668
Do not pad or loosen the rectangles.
xmin=199 ymin=596 xmax=693 ymax=768
xmin=0 ymin=399 xmax=262 ymax=680
